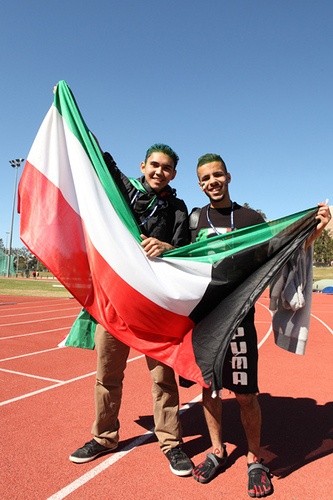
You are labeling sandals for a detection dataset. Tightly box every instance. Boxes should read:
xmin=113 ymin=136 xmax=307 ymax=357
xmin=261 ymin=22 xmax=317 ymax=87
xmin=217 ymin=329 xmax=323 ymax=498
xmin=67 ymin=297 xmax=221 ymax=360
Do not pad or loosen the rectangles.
xmin=246 ymin=458 xmax=273 ymax=498
xmin=192 ymin=446 xmax=227 ymax=483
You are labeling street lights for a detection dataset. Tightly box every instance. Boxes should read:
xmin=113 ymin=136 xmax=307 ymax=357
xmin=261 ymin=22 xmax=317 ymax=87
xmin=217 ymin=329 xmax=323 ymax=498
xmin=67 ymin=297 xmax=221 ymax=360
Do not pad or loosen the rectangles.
xmin=6 ymin=158 xmax=26 ymax=279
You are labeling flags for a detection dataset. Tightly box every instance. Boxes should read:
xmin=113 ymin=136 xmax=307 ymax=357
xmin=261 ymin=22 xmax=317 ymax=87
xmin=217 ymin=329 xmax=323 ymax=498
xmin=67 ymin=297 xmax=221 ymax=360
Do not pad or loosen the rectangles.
xmin=17 ymin=79 xmax=326 ymax=392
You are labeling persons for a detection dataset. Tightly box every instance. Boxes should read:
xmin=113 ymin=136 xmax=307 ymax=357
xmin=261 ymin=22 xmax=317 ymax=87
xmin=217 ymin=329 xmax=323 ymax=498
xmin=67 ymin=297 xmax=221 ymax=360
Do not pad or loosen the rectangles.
xmin=51 ymin=79 xmax=195 ymax=477
xmin=186 ymin=154 xmax=331 ymax=498
xmin=32 ymin=270 xmax=39 ymax=278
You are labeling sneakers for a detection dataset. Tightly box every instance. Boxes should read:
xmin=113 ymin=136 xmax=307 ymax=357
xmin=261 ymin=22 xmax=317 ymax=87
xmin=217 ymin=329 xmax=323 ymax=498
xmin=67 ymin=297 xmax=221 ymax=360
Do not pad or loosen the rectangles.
xmin=69 ymin=439 xmax=118 ymax=463
xmin=165 ymin=446 xmax=194 ymax=476
